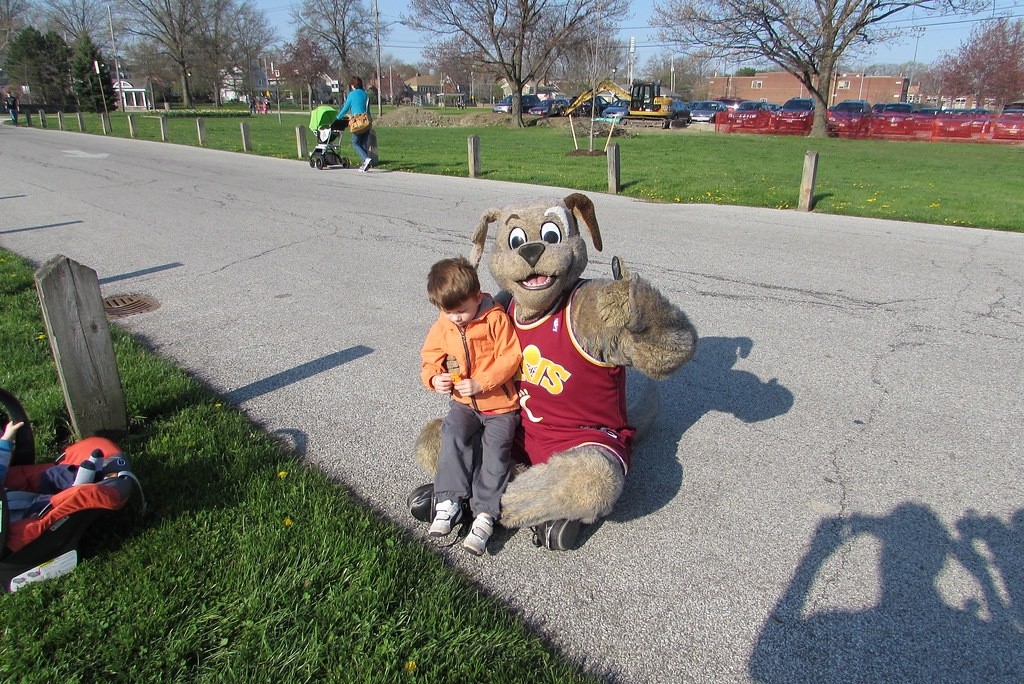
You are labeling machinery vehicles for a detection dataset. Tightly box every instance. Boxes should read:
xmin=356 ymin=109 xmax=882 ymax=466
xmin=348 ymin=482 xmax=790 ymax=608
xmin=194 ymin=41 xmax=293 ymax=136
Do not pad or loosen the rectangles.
xmin=561 ymin=77 xmax=692 ymax=129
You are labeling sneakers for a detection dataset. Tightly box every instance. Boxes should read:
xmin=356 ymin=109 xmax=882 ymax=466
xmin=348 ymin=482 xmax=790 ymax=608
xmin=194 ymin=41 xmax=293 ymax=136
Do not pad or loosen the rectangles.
xmin=430 ymin=499 xmax=465 ymax=537
xmin=463 ymin=513 xmax=493 ymax=558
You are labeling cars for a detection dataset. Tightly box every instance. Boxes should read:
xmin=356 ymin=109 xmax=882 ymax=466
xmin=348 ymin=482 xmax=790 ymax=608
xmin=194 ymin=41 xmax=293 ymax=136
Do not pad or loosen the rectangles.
xmin=492 ymin=95 xmax=540 ymax=113
xmin=826 ymin=106 xmax=837 ymax=122
xmin=914 ymin=108 xmax=942 ymax=129
xmin=828 ymin=99 xmax=872 ymax=130
xmin=876 ymin=103 xmax=918 ymax=131
xmin=731 ymin=101 xmax=772 ymax=127
xmin=528 ymin=100 xmax=569 ymax=116
xmin=968 ymin=109 xmax=995 ymax=132
xmin=931 ymin=109 xmax=972 ymax=137
xmin=774 ymin=97 xmax=815 ymax=126
xmin=991 ymin=100 xmax=1024 ymax=140
xmin=767 ymin=103 xmax=781 ymax=124
xmin=684 ymin=101 xmax=728 ymax=123
xmin=871 ymin=104 xmax=888 ymax=115
xmin=602 ymin=100 xmax=631 ymax=119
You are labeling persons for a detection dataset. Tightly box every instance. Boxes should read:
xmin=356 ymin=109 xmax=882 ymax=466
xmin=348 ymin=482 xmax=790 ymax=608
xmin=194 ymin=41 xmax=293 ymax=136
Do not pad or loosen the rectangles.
xmin=5 ymin=93 xmax=20 ymax=126
xmin=251 ymin=95 xmax=270 ymax=114
xmin=421 ymin=258 xmax=522 ymax=556
xmin=456 ymin=98 xmax=466 ymax=109
xmin=0 ymin=420 xmax=104 ymax=518
xmin=337 ymin=76 xmax=372 ymax=171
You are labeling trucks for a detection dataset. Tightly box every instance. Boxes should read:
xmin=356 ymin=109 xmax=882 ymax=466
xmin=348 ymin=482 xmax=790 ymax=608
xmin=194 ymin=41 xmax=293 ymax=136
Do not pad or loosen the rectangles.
xmin=715 ymin=97 xmax=749 ymax=120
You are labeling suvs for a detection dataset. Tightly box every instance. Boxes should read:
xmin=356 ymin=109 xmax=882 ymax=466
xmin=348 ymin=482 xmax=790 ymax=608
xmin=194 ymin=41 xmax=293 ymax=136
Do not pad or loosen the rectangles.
xmin=672 ymin=101 xmax=692 ymax=125
xmin=569 ymin=96 xmax=612 ymax=117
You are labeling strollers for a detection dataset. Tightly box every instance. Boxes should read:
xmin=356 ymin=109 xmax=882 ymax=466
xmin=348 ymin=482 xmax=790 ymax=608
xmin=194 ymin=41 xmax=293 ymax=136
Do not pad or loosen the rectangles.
xmin=308 ymin=105 xmax=350 ymax=170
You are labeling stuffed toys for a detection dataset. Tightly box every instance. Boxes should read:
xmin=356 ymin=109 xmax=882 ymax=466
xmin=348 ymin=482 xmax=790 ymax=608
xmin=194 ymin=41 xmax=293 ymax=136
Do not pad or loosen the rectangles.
xmin=408 ymin=194 xmax=697 ymax=551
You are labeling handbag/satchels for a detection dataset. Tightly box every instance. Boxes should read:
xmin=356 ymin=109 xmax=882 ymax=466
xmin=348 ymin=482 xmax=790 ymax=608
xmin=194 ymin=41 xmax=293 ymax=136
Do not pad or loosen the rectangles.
xmin=349 ymin=113 xmax=370 ymax=135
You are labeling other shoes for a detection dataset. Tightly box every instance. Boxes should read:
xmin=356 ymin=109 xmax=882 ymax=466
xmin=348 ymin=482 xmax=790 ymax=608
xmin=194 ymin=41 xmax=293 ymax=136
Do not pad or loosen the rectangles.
xmin=360 ymin=157 xmax=373 ymax=172
